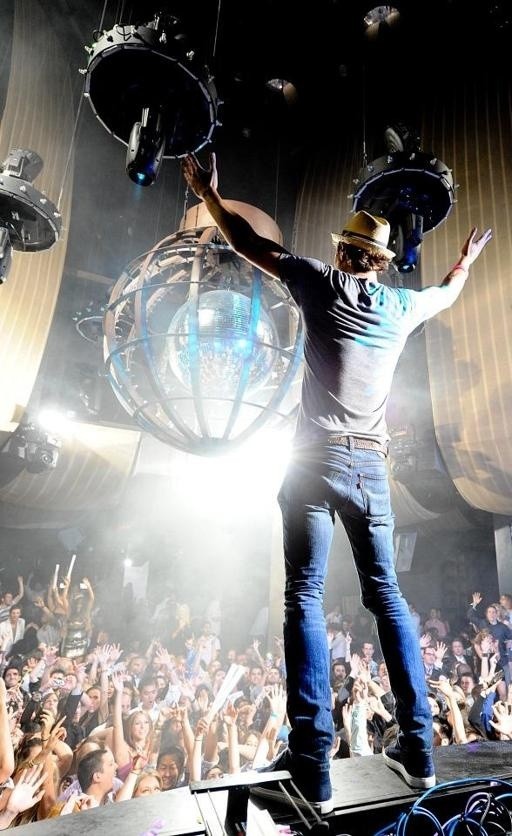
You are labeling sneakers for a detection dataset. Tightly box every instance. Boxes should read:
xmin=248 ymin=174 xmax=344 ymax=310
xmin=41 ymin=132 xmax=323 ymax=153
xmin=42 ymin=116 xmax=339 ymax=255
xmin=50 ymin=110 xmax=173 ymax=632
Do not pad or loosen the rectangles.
xmin=249 ymin=765 xmax=338 ymax=816
xmin=382 ymin=741 xmax=440 ymax=792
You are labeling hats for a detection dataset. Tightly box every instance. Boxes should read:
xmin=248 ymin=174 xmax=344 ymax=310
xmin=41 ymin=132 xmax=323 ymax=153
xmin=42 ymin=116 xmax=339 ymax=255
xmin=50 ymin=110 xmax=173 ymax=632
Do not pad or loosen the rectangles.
xmin=330 ymin=208 xmax=397 ymax=261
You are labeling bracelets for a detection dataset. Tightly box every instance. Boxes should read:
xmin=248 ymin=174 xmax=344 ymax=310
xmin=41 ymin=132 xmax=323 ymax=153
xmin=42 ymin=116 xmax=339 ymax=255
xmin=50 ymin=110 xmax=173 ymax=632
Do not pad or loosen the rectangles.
xmin=455 ymin=264 xmax=470 ymax=278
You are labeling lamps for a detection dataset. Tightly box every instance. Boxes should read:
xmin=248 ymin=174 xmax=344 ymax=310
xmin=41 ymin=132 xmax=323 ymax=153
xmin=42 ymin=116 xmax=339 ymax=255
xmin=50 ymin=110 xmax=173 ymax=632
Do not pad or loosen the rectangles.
xmin=347 ymin=112 xmax=461 ymax=277
xmin=1 ymin=0 xmax=107 ymax=282
xmin=78 ymin=0 xmax=226 ymax=187
xmin=103 ymin=156 xmax=309 ymax=458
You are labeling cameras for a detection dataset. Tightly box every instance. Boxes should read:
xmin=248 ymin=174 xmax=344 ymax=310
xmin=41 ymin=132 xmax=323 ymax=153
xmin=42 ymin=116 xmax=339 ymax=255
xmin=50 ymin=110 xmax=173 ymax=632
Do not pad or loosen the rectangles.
xmin=54 ymin=680 xmax=64 ymax=687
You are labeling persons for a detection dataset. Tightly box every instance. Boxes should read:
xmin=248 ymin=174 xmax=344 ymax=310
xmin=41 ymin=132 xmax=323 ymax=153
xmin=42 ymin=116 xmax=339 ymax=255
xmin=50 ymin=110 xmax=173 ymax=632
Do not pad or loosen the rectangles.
xmin=181 ymin=147 xmax=494 ymax=816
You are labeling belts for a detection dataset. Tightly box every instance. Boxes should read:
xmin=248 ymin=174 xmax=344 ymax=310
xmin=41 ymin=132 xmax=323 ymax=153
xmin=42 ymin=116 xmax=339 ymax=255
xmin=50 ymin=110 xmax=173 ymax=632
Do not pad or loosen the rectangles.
xmin=320 ymin=434 xmax=389 ymax=457
